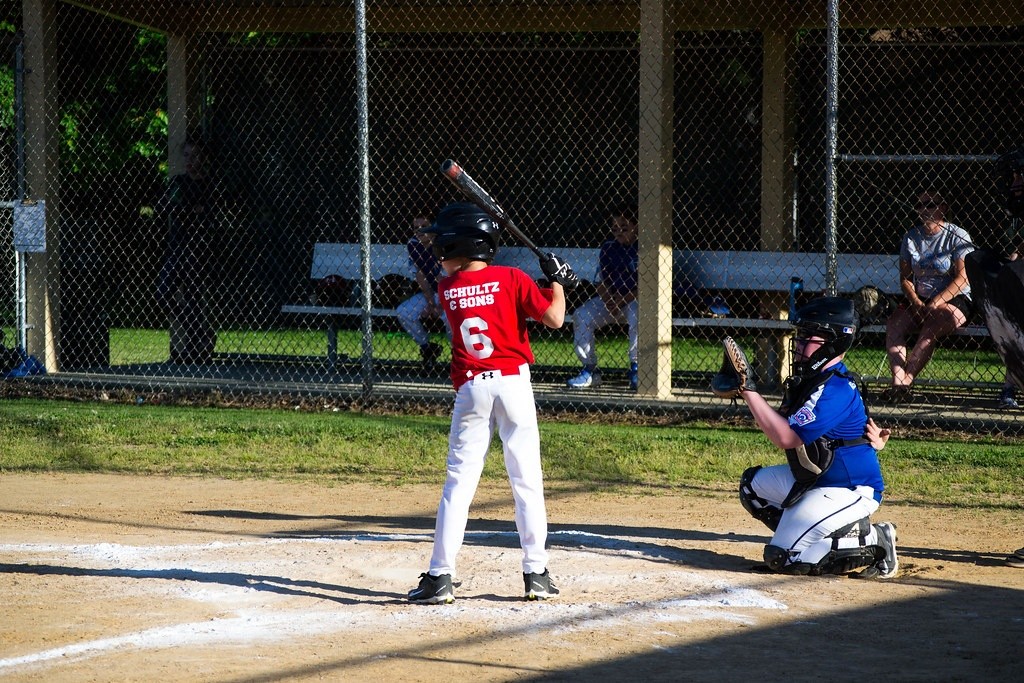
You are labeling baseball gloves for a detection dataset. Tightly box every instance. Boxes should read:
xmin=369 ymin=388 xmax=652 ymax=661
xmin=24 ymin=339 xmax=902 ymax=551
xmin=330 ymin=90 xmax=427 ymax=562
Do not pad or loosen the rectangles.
xmin=711 ymin=335 xmax=757 ymax=399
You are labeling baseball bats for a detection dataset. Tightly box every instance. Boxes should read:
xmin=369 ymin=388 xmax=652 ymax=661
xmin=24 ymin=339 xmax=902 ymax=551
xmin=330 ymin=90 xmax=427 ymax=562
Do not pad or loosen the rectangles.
xmin=440 ymin=159 xmax=580 ymax=290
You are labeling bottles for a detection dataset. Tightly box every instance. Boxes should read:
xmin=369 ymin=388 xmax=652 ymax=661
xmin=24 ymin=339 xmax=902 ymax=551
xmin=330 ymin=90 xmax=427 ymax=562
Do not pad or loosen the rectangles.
xmin=789 ymin=276 xmax=804 ymax=321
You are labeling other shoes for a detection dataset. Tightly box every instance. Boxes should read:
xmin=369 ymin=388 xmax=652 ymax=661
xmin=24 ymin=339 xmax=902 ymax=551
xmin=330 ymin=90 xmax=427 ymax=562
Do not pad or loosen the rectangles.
xmin=877 ymin=387 xmax=924 ymax=406
xmin=999 ymin=391 xmax=1018 ymax=409
xmin=419 ymin=342 xmax=443 ymax=376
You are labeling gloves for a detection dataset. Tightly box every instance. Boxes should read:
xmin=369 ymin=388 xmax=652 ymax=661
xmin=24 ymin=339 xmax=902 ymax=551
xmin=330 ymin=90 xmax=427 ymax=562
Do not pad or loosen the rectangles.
xmin=538 ymin=252 xmax=579 ymax=290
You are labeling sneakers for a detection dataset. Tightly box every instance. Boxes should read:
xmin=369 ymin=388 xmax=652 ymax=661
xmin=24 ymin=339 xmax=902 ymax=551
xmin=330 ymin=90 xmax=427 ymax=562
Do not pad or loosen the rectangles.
xmin=407 ymin=571 xmax=455 ymax=606
xmin=524 ymin=566 xmax=559 ymax=599
xmin=629 ymin=365 xmax=638 ymax=389
xmin=566 ymin=363 xmax=603 ymax=388
xmin=872 ymin=521 xmax=898 ymax=579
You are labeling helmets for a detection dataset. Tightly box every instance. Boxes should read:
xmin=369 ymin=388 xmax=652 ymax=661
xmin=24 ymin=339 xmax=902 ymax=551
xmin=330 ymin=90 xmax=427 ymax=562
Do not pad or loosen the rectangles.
xmin=783 ymin=300 xmax=862 ymax=391
xmin=419 ymin=202 xmax=502 ymax=262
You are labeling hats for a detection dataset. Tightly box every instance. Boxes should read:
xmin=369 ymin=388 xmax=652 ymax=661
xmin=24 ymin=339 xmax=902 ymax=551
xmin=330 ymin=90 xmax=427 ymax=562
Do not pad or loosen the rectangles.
xmin=915 ymin=195 xmax=947 ymax=220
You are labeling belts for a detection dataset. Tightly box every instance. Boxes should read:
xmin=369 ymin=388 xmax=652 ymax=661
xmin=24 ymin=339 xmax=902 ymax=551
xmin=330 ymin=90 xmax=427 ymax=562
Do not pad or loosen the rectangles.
xmin=470 ymin=367 xmax=521 ymax=379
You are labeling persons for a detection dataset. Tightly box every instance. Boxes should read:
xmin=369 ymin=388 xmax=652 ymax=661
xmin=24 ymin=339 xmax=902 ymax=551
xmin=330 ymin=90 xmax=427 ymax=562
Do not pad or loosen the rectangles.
xmin=972 ymin=247 xmax=1024 ymax=408
xmin=567 ymin=203 xmax=640 ymax=393
xmin=878 ymin=181 xmax=976 ymax=404
xmin=395 ymin=205 xmax=456 ymax=370
xmin=157 ymin=141 xmax=216 ymax=354
xmin=712 ymin=297 xmax=900 ymax=580
xmin=408 ymin=202 xmax=559 ymax=604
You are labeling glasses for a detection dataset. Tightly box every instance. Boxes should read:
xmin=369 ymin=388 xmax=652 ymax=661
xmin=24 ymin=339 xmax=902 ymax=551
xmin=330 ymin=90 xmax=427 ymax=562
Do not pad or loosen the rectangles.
xmin=610 ymin=227 xmax=629 ymax=233
xmin=413 ymin=225 xmax=426 ymax=232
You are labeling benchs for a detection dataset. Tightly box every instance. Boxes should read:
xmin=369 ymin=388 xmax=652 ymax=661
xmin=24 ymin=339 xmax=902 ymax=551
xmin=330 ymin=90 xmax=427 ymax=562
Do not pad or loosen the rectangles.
xmin=280 ymin=242 xmax=989 ymax=396
